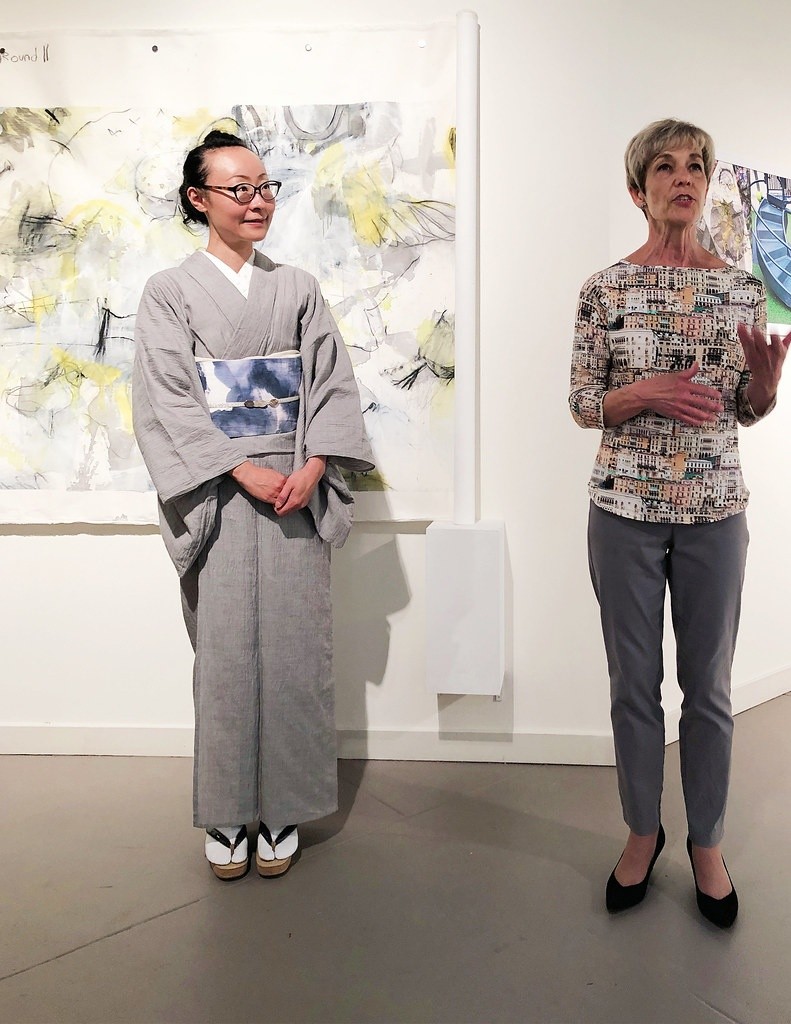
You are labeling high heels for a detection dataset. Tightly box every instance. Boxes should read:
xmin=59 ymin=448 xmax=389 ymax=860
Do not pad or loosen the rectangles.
xmin=205 ymin=824 xmax=249 ymax=879
xmin=684 ymin=833 xmax=738 ymax=930
xmin=605 ymin=826 xmax=665 ymax=916
xmin=255 ymin=818 xmax=298 ymax=876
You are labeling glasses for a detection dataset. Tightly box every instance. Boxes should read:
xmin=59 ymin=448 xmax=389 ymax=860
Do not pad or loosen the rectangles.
xmin=200 ymin=179 xmax=282 ymax=203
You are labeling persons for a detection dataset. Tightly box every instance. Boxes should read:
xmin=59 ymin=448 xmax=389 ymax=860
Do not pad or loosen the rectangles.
xmin=130 ymin=131 xmax=376 ymax=882
xmin=567 ymin=120 xmax=791 ymax=928
